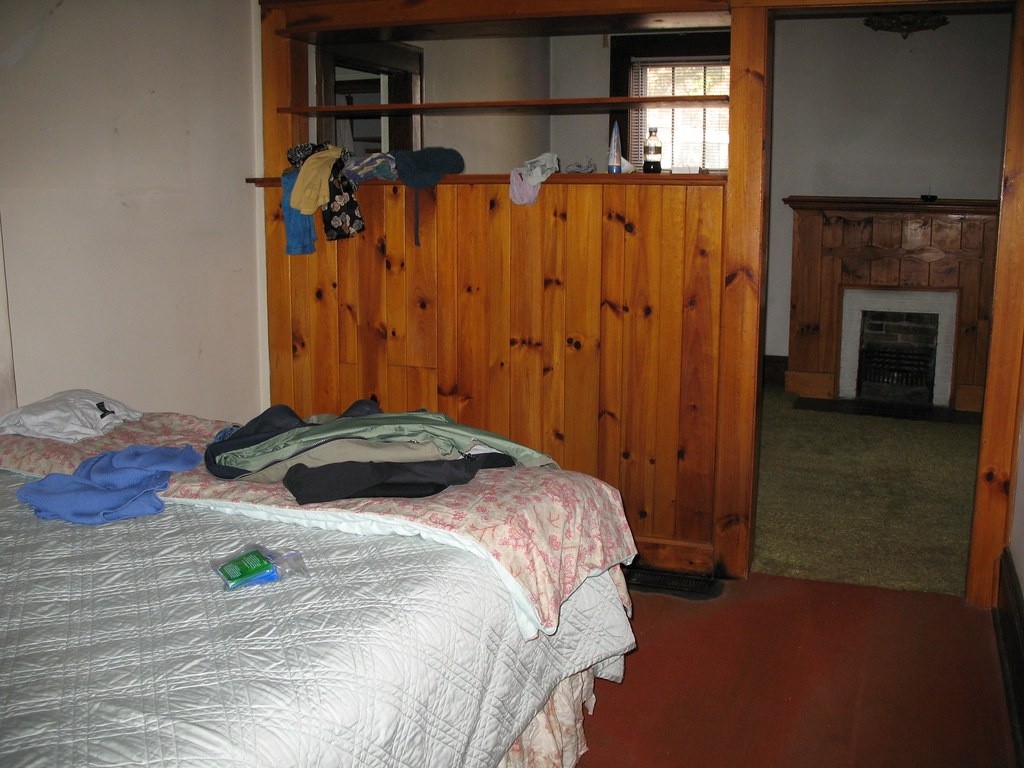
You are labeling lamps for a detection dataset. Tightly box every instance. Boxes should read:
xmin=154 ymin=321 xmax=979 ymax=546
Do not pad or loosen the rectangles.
xmin=864 ymin=12 xmax=951 ymax=39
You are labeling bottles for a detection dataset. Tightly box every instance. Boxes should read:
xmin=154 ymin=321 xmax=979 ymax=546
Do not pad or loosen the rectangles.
xmin=643 ymin=127 xmax=662 ymax=173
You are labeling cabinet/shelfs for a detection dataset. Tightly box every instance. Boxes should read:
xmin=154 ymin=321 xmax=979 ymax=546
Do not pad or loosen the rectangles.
xmin=783 ymin=194 xmax=1001 ymax=425
xmin=244 ymin=0 xmax=772 ymax=580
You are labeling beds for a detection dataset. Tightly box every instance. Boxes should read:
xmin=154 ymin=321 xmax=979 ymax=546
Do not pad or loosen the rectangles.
xmin=0 ymin=388 xmax=636 ymax=768
